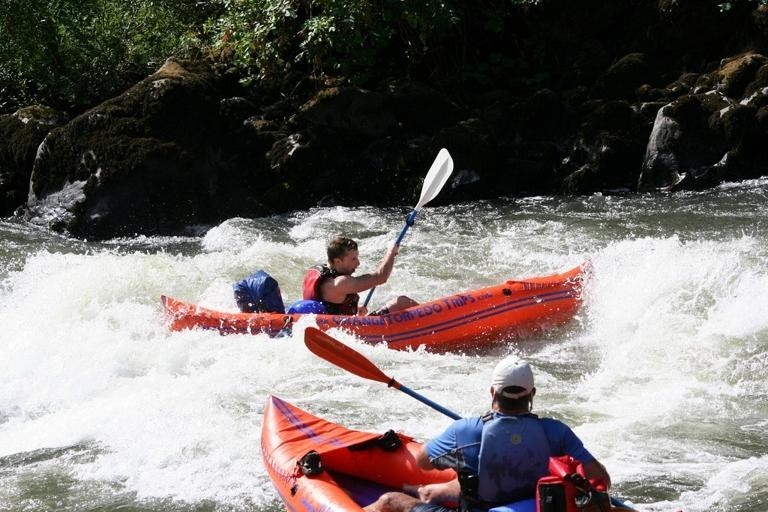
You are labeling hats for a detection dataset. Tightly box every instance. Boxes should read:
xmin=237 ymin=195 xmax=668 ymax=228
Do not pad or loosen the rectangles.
xmin=491 ymin=355 xmax=534 ymax=399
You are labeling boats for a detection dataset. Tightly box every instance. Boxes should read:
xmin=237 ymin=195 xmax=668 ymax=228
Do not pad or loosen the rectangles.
xmin=260 ymin=395 xmax=639 ymax=512
xmin=160 ymin=263 xmax=584 ymax=353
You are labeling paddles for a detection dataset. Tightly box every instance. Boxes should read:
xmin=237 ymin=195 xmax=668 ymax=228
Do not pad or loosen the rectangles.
xmin=360 ymin=148 xmax=454 ymax=314
xmin=304 ymin=327 xmax=625 ymax=505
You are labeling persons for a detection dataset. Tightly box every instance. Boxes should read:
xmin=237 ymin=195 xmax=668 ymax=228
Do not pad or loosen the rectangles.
xmin=301 ymin=237 xmax=419 ymax=316
xmin=361 ymin=354 xmax=611 ymax=512
